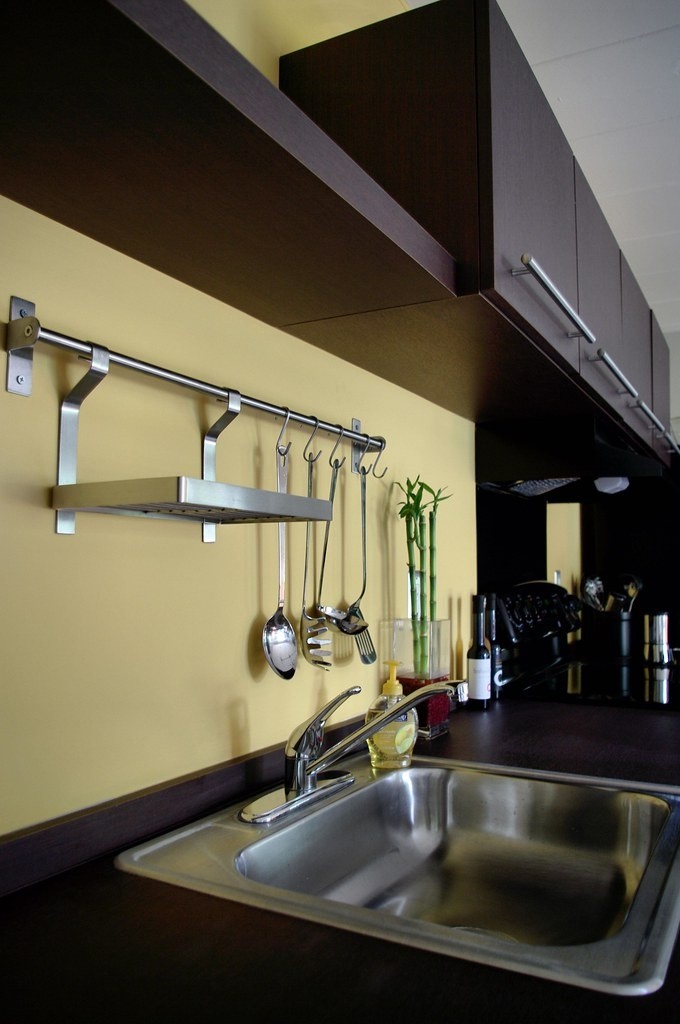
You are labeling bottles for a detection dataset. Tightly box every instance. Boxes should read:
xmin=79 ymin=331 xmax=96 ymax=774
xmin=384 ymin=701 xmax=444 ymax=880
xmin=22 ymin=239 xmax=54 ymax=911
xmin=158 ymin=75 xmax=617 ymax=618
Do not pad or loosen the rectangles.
xmin=485 ymin=593 xmax=502 ymax=701
xmin=467 ymin=596 xmax=492 ymax=710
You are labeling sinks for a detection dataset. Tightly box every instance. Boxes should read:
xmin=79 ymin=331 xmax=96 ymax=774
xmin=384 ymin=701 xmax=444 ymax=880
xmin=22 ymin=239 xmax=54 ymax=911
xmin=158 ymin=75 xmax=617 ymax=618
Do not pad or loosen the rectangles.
xmin=114 ymin=754 xmax=680 ymax=996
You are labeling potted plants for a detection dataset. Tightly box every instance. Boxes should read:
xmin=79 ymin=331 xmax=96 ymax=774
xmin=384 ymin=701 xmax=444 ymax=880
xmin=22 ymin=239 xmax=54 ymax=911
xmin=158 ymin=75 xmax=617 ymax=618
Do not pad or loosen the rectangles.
xmin=374 ymin=477 xmax=456 ymax=740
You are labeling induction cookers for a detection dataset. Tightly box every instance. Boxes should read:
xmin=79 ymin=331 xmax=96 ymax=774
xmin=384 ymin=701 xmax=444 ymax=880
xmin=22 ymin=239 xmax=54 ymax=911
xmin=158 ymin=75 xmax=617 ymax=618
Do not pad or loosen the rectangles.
xmin=495 ymin=579 xmax=680 ymax=713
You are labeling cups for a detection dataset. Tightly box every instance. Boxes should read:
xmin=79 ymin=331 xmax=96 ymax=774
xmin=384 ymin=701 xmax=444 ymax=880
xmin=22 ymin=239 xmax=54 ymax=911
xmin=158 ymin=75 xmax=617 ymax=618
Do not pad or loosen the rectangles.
xmin=586 ymin=607 xmax=634 ymax=659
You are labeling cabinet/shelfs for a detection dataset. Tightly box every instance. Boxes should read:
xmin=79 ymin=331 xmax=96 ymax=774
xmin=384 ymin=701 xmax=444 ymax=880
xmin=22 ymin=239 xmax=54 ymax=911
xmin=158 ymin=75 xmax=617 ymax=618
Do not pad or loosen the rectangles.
xmin=280 ymin=0 xmax=672 ymax=481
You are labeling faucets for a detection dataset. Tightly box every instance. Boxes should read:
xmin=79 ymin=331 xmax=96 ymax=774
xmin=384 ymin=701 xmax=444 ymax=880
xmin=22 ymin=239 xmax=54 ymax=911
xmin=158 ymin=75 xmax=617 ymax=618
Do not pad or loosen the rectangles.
xmin=282 ymin=680 xmax=469 ymax=793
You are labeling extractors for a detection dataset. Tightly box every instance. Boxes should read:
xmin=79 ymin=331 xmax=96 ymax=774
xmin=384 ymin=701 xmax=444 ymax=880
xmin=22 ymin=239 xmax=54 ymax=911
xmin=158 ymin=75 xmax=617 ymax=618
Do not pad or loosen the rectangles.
xmin=476 ymin=398 xmax=668 ymax=502
